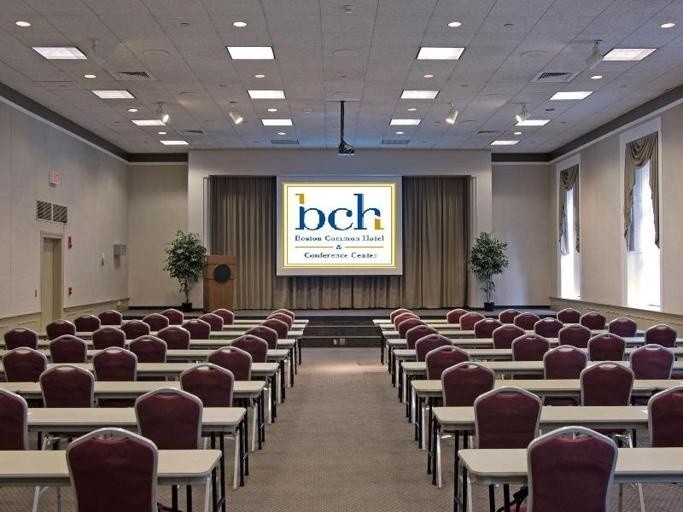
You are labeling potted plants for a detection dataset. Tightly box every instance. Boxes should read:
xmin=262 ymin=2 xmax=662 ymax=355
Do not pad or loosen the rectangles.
xmin=164 ymin=230 xmax=207 ymax=311
xmin=466 ymin=230 xmax=509 ymax=310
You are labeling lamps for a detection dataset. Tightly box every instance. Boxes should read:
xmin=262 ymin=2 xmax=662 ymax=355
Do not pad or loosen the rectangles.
xmin=585 ymin=40 xmax=603 ymax=70
xmin=229 ymin=102 xmax=243 ymax=124
xmin=156 ymin=101 xmax=169 ymax=123
xmin=337 ymin=100 xmax=356 ymax=154
xmin=515 ymin=104 xmax=528 ymax=124
xmin=446 ymin=102 xmax=459 ymax=124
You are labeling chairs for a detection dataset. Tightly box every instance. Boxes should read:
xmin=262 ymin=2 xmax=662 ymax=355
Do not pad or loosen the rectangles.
xmin=525 ymin=426 xmax=619 ymax=511
xmin=133 ymin=387 xmax=219 ymax=511
xmin=179 ymin=364 xmax=243 ymax=489
xmin=205 ymin=345 xmax=263 ymax=453
xmin=0 ymin=388 xmax=49 ymax=511
xmin=646 ymin=386 xmax=683 ymax=447
xmin=472 ymin=385 xmax=544 ymax=511
xmin=40 ymin=365 xmax=94 ymax=451
xmin=435 ymin=361 xmax=495 ymax=490
xmin=575 ymin=362 xmax=648 ymax=509
xmin=1 ymin=308 xmax=307 ymax=422
xmin=419 ymin=346 xmax=472 ymax=449
xmin=66 ymin=427 xmax=168 ymax=510
xmin=375 ymin=309 xmax=682 ymax=407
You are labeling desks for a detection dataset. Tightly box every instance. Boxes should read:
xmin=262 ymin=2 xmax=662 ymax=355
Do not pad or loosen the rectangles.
xmin=26 ymin=407 xmax=250 ymax=489
xmin=457 ymin=447 xmax=682 ymax=511
xmin=410 ymin=380 xmax=682 ymax=450
xmin=1 ymin=449 xmax=226 ymax=510
xmin=431 ymin=406 xmax=648 ymax=487
xmin=2 ymin=379 xmax=267 ymax=451
